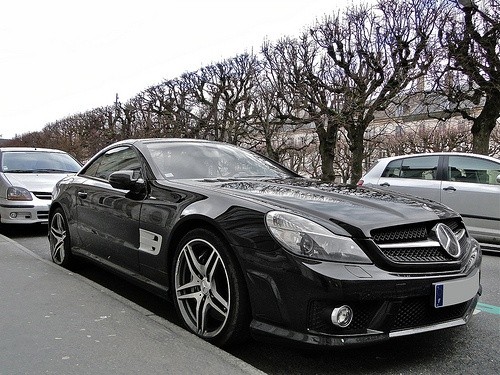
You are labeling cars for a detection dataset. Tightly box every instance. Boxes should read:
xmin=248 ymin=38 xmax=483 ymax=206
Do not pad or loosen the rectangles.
xmin=46 ymin=138 xmax=482 ymax=351
xmin=0 ymin=147 xmax=83 ymax=227
xmin=357 ymin=154 xmax=500 ymax=251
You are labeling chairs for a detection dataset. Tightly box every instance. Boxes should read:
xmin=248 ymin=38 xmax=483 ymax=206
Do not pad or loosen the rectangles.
xmin=450 ymin=168 xmax=470 ymax=183
